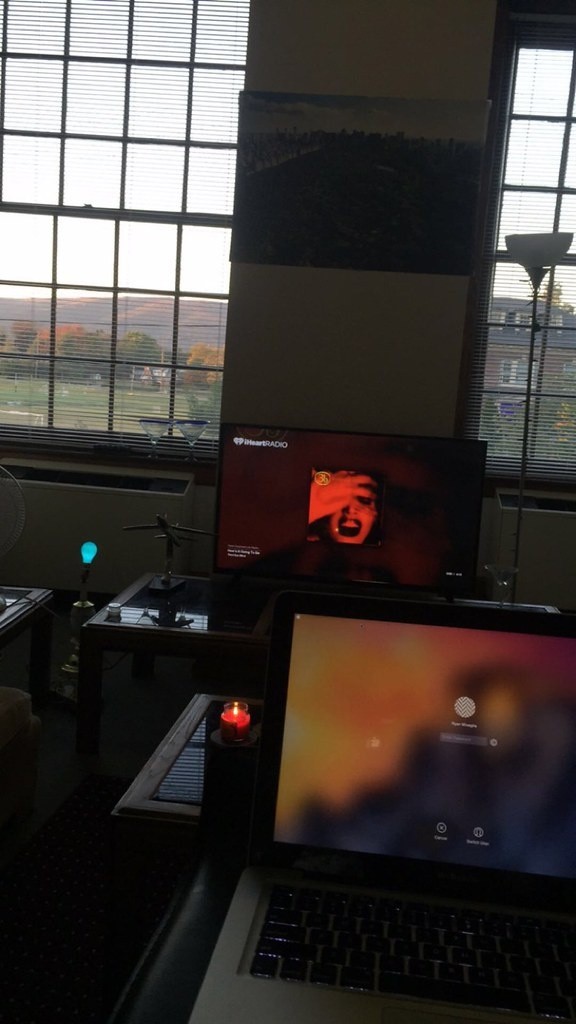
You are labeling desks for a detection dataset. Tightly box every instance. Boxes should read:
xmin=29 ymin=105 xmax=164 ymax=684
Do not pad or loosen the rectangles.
xmin=111 ymin=693 xmax=265 ymax=826
xmin=0 ymin=585 xmax=54 ymax=705
xmin=73 ymin=573 xmax=286 ymax=754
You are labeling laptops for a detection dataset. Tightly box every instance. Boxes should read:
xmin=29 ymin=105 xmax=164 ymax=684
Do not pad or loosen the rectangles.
xmin=189 ymin=590 xmax=576 ymax=1024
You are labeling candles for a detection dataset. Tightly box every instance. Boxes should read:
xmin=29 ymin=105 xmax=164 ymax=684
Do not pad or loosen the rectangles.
xmin=220 ymin=700 xmax=251 ymax=743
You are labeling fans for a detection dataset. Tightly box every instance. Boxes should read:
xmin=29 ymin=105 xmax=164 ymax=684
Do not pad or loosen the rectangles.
xmin=0 ymin=466 xmax=27 ymax=559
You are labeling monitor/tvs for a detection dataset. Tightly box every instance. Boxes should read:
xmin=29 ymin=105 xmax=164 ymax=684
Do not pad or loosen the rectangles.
xmin=212 ymin=423 xmax=488 ymax=598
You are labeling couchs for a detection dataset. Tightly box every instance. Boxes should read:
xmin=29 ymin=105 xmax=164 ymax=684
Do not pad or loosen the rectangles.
xmin=0 ymin=687 xmax=42 ymax=828
